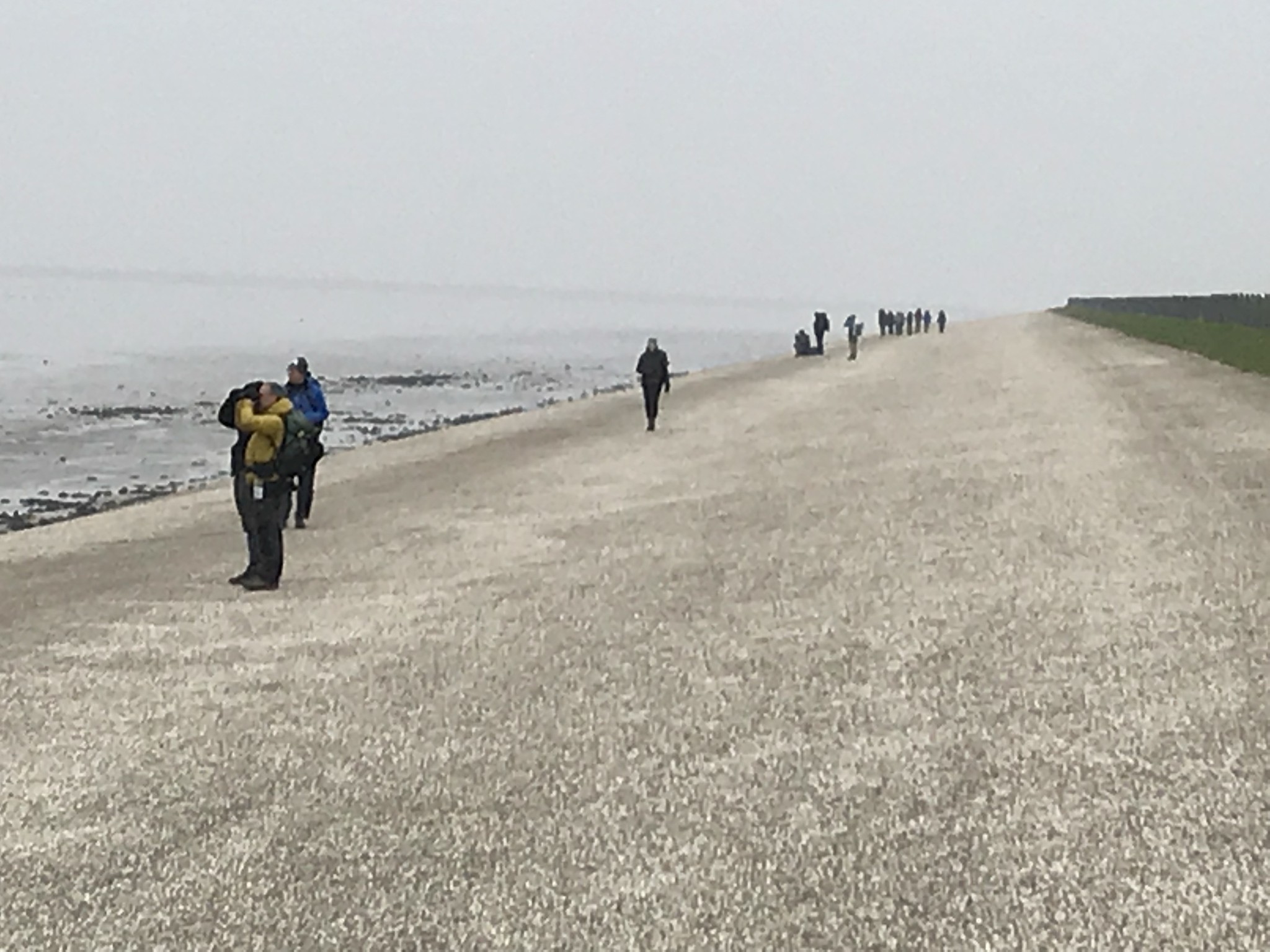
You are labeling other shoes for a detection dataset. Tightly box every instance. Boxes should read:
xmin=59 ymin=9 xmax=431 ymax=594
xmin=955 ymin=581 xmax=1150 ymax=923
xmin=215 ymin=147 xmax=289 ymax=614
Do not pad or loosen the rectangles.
xmin=647 ymin=420 xmax=655 ymax=431
xmin=228 ymin=571 xmax=251 ymax=585
xmin=848 ymin=356 xmax=855 ymax=361
xmin=294 ymin=515 xmax=305 ymax=529
xmin=239 ymin=576 xmax=277 ymax=591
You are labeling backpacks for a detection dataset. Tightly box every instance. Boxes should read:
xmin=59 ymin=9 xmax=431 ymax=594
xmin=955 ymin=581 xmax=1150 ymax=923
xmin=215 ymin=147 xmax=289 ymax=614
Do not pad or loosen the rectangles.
xmin=276 ymin=410 xmax=314 ymax=476
xmin=856 ymin=322 xmax=863 ymax=335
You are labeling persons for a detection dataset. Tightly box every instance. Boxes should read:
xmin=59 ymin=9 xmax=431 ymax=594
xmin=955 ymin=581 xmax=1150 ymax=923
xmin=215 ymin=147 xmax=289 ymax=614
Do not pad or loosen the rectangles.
xmin=877 ymin=308 xmax=947 ymax=338
xmin=842 ymin=315 xmax=865 ymax=361
xmin=793 ymin=312 xmax=830 ymax=357
xmin=218 ymin=357 xmax=329 ymax=593
xmin=635 ymin=337 xmax=670 ymax=433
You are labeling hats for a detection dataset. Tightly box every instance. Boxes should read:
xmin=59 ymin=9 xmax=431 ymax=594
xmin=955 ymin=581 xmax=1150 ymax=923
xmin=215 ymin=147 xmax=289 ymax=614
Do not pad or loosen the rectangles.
xmin=288 ymin=356 xmax=308 ymax=373
